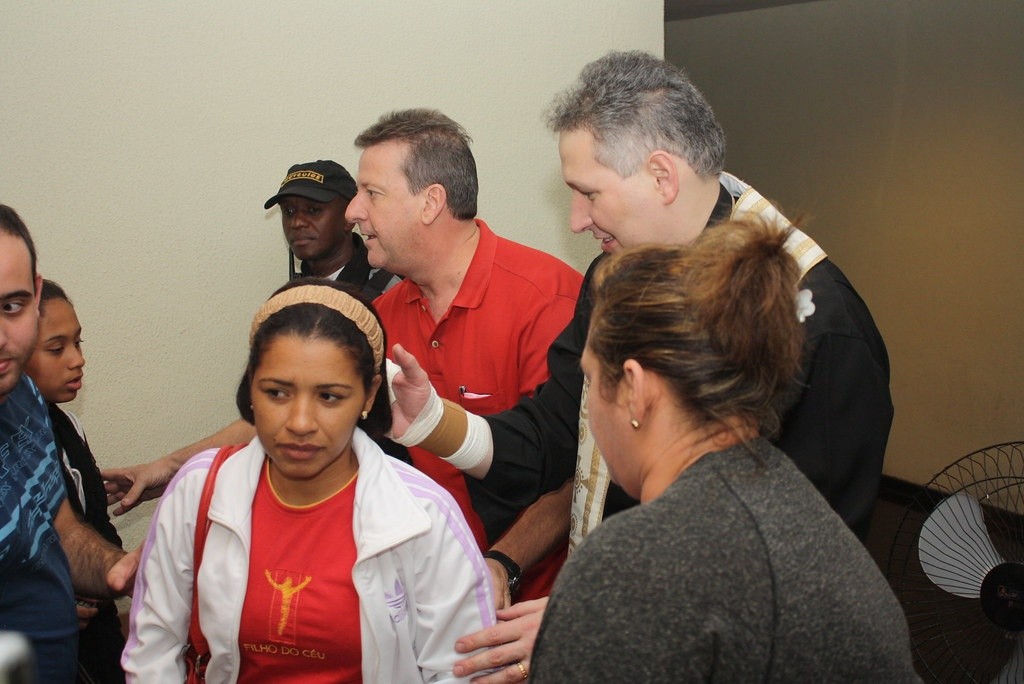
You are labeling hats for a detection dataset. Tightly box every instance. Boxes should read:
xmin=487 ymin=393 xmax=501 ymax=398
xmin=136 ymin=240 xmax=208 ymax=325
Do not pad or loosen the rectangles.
xmin=264 ymin=160 xmax=357 ymax=210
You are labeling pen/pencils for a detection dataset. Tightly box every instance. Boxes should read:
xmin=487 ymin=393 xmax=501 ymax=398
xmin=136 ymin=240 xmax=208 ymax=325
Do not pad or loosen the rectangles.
xmin=458 ymin=385 xmax=467 ymax=394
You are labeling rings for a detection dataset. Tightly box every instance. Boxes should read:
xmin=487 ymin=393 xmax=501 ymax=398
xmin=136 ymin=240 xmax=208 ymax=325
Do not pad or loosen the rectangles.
xmin=517 ymin=662 xmax=527 ymax=678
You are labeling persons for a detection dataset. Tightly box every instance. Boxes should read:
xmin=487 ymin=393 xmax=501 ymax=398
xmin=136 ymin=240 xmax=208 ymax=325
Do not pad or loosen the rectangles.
xmin=265 ymin=159 xmax=407 ymax=304
xmin=529 ymin=217 xmax=922 ymax=684
xmin=102 ymin=109 xmax=584 ymax=611
xmin=0 ymin=204 xmax=146 ymax=684
xmin=388 ymin=58 xmax=893 ymax=684
xmin=120 ymin=280 xmax=502 ymax=684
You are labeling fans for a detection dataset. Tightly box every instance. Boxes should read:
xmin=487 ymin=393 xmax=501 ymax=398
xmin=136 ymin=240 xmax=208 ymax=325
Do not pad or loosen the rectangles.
xmin=887 ymin=441 xmax=1023 ymax=684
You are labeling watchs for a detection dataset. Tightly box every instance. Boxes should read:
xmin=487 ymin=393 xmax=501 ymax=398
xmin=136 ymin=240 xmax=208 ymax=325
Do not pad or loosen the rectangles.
xmin=483 ymin=550 xmax=525 ymax=594
